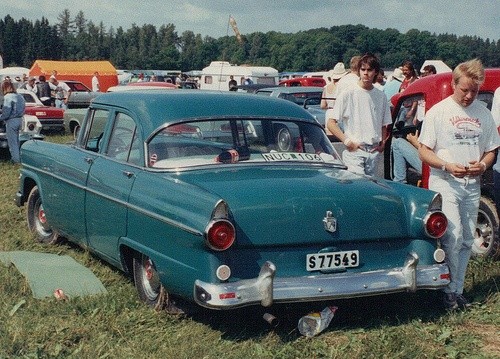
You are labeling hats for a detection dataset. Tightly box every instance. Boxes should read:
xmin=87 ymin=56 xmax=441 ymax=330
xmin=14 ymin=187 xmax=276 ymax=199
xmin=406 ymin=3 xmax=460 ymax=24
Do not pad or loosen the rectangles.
xmin=28 ymin=77 xmax=36 ymax=81
xmin=331 ymin=62 xmax=349 ymax=79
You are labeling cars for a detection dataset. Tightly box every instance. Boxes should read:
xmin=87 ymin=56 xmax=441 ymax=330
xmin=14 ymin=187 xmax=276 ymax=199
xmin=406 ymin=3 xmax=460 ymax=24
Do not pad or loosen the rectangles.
xmin=13 ymin=89 xmax=451 ymax=314
xmin=62 ymin=113 xmax=259 ymax=158
xmin=229 ymin=70 xmax=344 ymax=160
xmin=382 ymin=68 xmax=500 ymax=262
xmin=2 ymin=80 xmax=105 ymax=136
xmin=107 ymin=72 xmax=200 ymax=91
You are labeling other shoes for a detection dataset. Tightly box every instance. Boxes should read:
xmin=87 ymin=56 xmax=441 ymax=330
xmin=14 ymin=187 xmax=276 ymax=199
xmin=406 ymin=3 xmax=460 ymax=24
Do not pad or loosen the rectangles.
xmin=437 ymin=292 xmax=471 ymax=314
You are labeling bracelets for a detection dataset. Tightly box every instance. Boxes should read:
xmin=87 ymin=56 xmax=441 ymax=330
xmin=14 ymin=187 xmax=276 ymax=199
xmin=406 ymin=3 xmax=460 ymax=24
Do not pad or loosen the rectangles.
xmin=344 ymin=138 xmax=351 ymax=145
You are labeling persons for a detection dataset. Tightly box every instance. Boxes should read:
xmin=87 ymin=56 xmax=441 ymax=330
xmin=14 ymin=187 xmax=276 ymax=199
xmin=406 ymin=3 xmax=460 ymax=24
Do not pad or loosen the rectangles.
xmin=327 ymin=55 xmax=392 ymax=176
xmin=0 ymin=79 xmax=25 ymax=164
xmin=279 ymin=76 xmax=307 ymax=86
xmin=393 ymin=130 xmax=424 ymax=184
xmin=34 ymin=76 xmax=51 ymax=107
xmin=228 ymin=75 xmax=237 ymax=91
xmin=241 ymin=78 xmax=253 ymax=84
xmin=131 ymin=72 xmax=155 ymax=82
xmin=321 ymin=57 xmax=436 ymax=135
xmin=418 ymin=59 xmax=500 ymax=308
xmin=92 ymin=72 xmax=101 ymax=91
xmin=5 ymin=73 xmax=36 ymax=91
xmin=50 ymin=76 xmax=71 ymax=109
xmin=176 ymin=74 xmax=201 ymax=89
xmin=51 ymin=71 xmax=57 ymax=78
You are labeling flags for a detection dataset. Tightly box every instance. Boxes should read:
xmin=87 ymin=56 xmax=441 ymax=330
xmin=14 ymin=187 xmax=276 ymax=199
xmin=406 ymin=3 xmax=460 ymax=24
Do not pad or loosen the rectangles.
xmin=230 ymin=17 xmax=241 ymax=41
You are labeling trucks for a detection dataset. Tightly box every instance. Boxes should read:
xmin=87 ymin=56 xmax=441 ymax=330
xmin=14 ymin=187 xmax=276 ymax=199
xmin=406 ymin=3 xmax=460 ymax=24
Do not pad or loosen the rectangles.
xmin=196 ymin=61 xmax=279 ymax=93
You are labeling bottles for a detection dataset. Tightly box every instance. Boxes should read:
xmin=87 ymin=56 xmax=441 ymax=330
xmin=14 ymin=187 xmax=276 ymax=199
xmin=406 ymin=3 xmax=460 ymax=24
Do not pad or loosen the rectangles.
xmin=215 ymin=147 xmax=251 ymax=164
